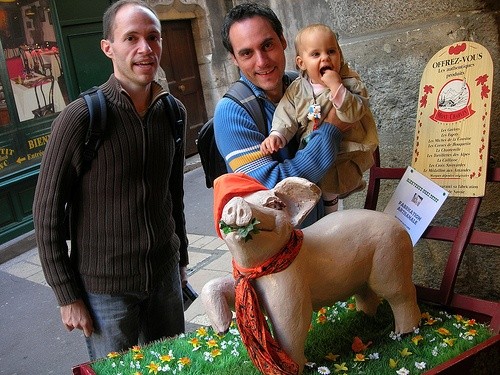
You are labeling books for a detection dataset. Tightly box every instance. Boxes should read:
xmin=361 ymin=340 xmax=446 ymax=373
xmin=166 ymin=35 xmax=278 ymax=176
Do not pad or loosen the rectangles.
xmin=182 ymin=282 xmax=198 ymax=312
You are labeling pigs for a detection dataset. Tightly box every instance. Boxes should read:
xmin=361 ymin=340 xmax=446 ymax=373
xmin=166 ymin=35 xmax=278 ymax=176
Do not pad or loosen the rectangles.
xmin=201 ymin=173 xmax=421 ymax=374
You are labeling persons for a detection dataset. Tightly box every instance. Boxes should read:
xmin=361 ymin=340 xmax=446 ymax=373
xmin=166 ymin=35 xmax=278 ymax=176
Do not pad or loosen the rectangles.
xmin=213 ymin=1 xmax=358 ymax=230
xmin=260 ymin=23 xmax=380 ymax=216
xmin=32 ymin=0 xmax=189 ymax=362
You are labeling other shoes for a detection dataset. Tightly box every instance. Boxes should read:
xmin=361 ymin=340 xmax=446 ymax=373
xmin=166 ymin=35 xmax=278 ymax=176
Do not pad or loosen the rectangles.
xmin=336 ymin=180 xmax=366 ymax=201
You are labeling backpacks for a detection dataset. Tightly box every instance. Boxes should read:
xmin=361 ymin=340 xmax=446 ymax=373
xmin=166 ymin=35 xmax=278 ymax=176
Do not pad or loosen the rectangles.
xmin=197 ymin=77 xmax=279 ymax=189
